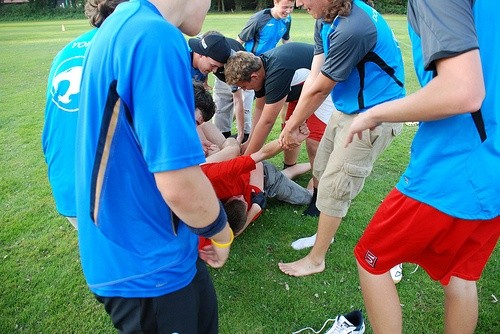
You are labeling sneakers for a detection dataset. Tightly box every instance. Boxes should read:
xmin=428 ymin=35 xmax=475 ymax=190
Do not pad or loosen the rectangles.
xmin=390 ymin=263 xmax=403 ymax=284
xmin=292 ymin=309 xmax=366 ymax=334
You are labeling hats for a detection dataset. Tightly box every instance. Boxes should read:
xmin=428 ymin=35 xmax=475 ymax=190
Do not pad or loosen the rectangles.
xmin=188 ymin=30 xmax=231 ymax=64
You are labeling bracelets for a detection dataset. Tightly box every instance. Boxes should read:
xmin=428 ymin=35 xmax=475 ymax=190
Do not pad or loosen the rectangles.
xmin=211 ymin=226 xmax=235 ymax=248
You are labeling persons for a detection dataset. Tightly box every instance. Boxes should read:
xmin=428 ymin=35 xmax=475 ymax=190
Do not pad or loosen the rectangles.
xmin=76 ymin=0 xmax=236 ymax=334
xmin=223 ymin=42 xmax=336 ymax=217
xmin=196 ymin=29 xmax=256 ymax=145
xmin=193 ymin=119 xmax=315 ymax=251
xmin=237 ymin=0 xmax=297 ymax=56
xmin=190 ymin=82 xmax=217 ymax=130
xmin=43 ymin=0 xmax=131 ymax=232
xmin=185 ymin=30 xmax=231 ymax=91
xmin=352 ymin=0 xmax=500 ymax=334
xmin=278 ymin=0 xmax=405 ymax=279
